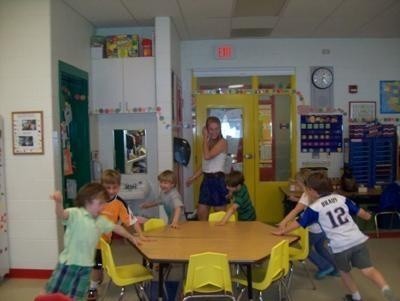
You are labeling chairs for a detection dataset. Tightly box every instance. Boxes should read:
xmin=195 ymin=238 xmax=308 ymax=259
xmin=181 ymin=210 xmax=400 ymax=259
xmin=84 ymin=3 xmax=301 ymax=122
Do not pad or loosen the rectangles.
xmin=143 ymin=218 xmax=165 ymax=230
xmin=100 ymin=239 xmax=152 ymax=301
xmin=366 ymin=183 xmax=400 ymax=238
xmin=260 ymin=221 xmax=316 ymax=294
xmin=183 ymin=252 xmax=236 ymax=301
xmin=208 ymin=211 xmax=235 ymax=220
xmin=231 ymin=240 xmax=294 ymax=301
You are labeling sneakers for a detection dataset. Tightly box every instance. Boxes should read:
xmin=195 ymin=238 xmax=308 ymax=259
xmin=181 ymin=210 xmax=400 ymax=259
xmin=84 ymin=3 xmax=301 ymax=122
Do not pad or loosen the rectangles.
xmin=316 ymin=266 xmax=333 ymax=279
xmin=88 ymin=290 xmax=99 ymax=301
xmin=344 ymin=295 xmax=362 ymax=301
xmin=329 ymin=268 xmax=339 ymax=276
xmin=383 ymin=289 xmax=396 ymax=301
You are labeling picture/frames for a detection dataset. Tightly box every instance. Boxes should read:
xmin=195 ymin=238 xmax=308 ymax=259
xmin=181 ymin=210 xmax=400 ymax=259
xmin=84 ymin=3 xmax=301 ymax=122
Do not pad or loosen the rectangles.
xmin=12 ymin=111 xmax=43 ymax=155
xmin=378 ymin=80 xmax=400 ymax=114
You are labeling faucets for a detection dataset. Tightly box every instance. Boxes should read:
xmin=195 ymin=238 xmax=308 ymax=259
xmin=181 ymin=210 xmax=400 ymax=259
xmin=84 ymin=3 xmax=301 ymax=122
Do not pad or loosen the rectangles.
xmin=128 ymin=184 xmax=132 ymax=189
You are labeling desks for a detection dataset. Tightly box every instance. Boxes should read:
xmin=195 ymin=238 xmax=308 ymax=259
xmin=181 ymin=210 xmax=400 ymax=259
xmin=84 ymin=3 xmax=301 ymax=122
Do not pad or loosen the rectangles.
xmin=280 ymin=183 xmax=385 ymax=227
xmin=131 ymin=221 xmax=299 ymax=301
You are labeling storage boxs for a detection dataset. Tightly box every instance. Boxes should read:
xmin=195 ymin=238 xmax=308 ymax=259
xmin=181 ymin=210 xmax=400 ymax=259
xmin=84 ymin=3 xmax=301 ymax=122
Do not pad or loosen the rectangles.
xmin=105 ymin=33 xmax=138 ymax=57
xmin=90 ymin=35 xmax=104 ymax=47
xmin=90 ymin=46 xmax=103 ymax=58
xmin=142 ymin=39 xmax=152 ymax=56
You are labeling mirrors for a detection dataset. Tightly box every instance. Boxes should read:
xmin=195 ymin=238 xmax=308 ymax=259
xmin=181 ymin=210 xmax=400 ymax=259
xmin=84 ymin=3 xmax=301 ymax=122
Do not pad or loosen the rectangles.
xmin=113 ymin=128 xmax=146 ymax=175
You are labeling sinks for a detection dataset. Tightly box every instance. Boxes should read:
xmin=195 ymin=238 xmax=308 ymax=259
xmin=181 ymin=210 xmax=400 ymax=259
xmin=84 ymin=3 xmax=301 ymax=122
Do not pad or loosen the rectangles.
xmin=117 ymin=182 xmax=150 ymax=201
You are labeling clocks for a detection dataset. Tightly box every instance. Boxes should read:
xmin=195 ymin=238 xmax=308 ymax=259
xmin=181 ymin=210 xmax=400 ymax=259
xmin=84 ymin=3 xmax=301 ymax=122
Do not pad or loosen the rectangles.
xmin=312 ymin=67 xmax=333 ymax=108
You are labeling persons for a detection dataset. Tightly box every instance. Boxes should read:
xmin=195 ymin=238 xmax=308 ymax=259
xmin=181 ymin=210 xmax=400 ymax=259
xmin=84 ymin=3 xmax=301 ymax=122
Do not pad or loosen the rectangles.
xmin=218 ymin=165 xmax=256 ymax=224
xmin=46 ymin=181 xmax=142 ymax=298
xmin=340 ymin=165 xmax=356 ymax=193
xmin=276 ymin=172 xmax=392 ymax=301
xmin=274 ymin=167 xmax=334 ymax=280
xmin=137 ymin=170 xmax=186 ymax=229
xmin=51 ymin=167 xmax=156 ymax=294
xmin=185 ymin=115 xmax=235 ymax=222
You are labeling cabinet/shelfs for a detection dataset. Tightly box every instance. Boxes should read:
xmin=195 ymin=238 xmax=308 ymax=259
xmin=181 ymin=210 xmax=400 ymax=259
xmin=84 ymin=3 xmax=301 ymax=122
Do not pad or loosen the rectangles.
xmin=91 ymin=58 xmax=158 ymax=115
